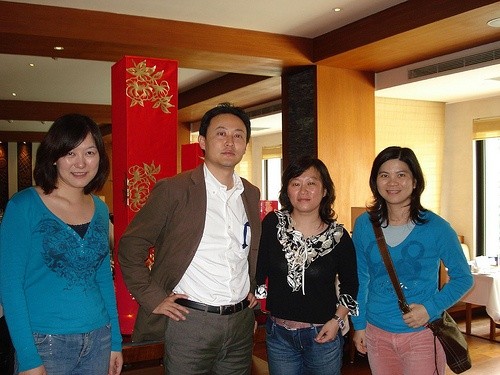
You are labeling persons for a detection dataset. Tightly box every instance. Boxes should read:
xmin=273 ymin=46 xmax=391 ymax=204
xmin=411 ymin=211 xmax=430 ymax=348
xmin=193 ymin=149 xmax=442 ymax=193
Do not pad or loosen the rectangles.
xmin=255 ymin=156 xmax=359 ymax=375
xmin=0 ymin=113 xmax=124 ymax=375
xmin=351 ymin=146 xmax=474 ymax=375
xmin=117 ymin=102 xmax=262 ymax=375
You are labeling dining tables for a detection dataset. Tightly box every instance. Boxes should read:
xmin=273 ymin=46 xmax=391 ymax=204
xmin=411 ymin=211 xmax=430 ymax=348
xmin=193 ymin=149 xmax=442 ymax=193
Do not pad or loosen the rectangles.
xmin=446 ymin=256 xmax=500 ymax=343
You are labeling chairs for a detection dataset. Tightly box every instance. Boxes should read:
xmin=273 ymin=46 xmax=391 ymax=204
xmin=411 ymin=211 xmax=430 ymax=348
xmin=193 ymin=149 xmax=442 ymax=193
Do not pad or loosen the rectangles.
xmin=438 ymin=244 xmax=480 ymax=313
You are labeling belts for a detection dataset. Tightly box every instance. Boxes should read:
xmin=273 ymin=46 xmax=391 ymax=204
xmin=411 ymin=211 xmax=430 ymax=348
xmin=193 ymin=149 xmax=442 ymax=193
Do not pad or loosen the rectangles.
xmin=267 ymin=310 xmax=325 ymax=331
xmin=173 ymin=293 xmax=250 ymax=316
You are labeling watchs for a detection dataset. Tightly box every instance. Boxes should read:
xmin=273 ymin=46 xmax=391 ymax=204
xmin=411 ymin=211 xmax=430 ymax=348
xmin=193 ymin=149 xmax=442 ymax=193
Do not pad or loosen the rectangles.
xmin=333 ymin=315 xmax=346 ymax=329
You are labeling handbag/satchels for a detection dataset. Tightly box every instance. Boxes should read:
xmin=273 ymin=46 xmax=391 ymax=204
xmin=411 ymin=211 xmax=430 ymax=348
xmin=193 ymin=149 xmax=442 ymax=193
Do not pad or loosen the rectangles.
xmin=427 ymin=310 xmax=472 ymax=375
